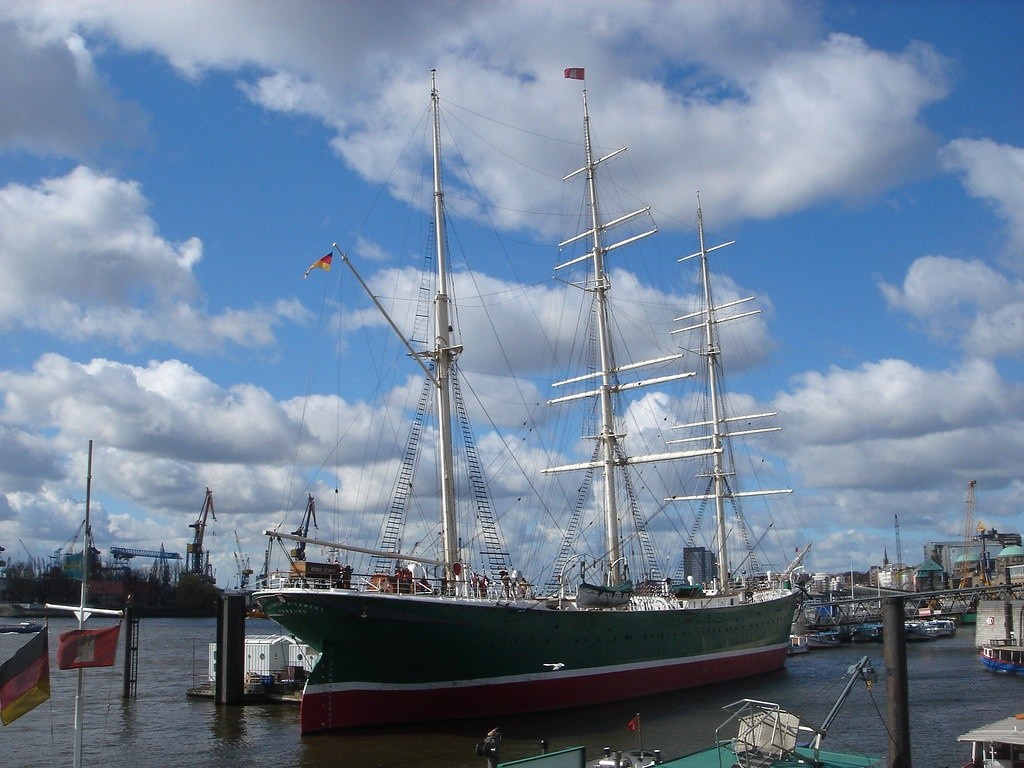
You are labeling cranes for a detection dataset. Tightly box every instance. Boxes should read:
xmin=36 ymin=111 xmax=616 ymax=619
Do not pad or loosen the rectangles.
xmin=184 ymin=487 xmax=220 ymax=577
xmin=17 ymin=521 xmax=104 ymax=579
xmin=232 ymin=529 xmax=254 ymax=590
xmin=109 ymin=546 xmax=183 ymax=579
xmin=289 ymin=492 xmax=319 ymax=561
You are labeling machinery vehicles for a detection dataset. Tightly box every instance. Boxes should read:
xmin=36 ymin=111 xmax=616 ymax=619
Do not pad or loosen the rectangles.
xmin=955 ymin=478 xmax=979 ymax=601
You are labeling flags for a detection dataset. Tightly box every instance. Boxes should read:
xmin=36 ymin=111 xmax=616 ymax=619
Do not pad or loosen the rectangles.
xmin=627 ymin=716 xmax=638 ymax=734
xmin=304 ymin=252 xmax=332 ymax=279
xmin=0 ymin=627 xmax=50 ymax=727
xmin=56 ymin=623 xmax=120 ymax=671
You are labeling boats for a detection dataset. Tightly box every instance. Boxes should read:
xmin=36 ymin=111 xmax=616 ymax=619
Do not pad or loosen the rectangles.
xmin=0 ymin=621 xmax=43 ymax=634
xmin=974 ymin=638 xmax=1024 ymax=676
xmin=954 ymin=712 xmax=1024 ymax=768
xmin=577 ymin=560 xmax=634 ymax=606
xmin=674 ymin=583 xmax=704 ymax=596
xmin=471 ymin=653 xmax=891 ymax=768
xmin=785 ymin=615 xmax=957 ymax=656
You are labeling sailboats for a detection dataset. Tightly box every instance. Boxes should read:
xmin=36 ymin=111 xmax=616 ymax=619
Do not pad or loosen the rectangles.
xmin=248 ymin=63 xmax=811 ymax=740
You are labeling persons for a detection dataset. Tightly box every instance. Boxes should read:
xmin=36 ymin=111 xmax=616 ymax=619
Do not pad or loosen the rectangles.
xmin=471 ymin=570 xmax=535 ymax=599
